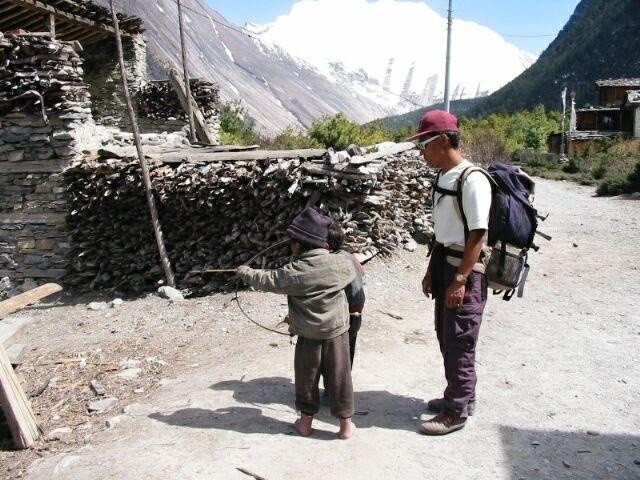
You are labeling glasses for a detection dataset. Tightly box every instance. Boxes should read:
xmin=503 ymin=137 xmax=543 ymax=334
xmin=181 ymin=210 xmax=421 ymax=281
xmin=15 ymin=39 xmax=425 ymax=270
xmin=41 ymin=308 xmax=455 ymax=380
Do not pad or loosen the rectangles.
xmin=416 ymin=135 xmax=441 ymax=150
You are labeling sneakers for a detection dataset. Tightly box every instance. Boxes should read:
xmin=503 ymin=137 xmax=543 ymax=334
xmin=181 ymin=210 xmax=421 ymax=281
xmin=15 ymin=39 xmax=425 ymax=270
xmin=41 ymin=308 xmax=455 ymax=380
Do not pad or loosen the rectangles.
xmin=429 ymin=398 xmax=475 ymax=416
xmin=420 ymin=412 xmax=467 ymax=435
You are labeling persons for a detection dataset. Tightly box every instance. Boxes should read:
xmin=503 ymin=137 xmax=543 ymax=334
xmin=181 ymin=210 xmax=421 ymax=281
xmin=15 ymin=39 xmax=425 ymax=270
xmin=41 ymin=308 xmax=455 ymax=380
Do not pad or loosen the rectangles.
xmin=281 ymin=225 xmax=366 ymax=399
xmin=404 ymin=108 xmax=492 ymax=436
xmin=235 ymin=205 xmax=357 ymax=441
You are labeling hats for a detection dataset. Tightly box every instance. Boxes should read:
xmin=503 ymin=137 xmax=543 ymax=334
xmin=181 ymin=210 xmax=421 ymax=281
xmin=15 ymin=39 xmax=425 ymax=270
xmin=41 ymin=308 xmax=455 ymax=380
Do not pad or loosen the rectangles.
xmin=286 ymin=207 xmax=332 ymax=249
xmin=406 ymin=110 xmax=457 ymax=142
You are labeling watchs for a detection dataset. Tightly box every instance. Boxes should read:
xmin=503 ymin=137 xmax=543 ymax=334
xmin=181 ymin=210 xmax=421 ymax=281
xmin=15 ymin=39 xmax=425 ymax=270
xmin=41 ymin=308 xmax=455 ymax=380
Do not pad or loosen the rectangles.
xmin=454 ymin=273 xmax=467 ymax=284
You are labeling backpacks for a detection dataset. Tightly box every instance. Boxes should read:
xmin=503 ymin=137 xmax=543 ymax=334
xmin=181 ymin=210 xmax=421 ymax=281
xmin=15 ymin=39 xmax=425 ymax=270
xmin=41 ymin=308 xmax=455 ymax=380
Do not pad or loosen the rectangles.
xmin=432 ymin=162 xmax=538 ymax=251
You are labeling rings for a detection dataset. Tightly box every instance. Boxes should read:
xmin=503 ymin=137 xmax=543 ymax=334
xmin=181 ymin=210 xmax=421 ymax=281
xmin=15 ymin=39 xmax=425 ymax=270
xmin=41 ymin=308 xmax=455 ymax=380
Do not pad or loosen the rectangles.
xmin=456 ymin=304 xmax=460 ymax=307
